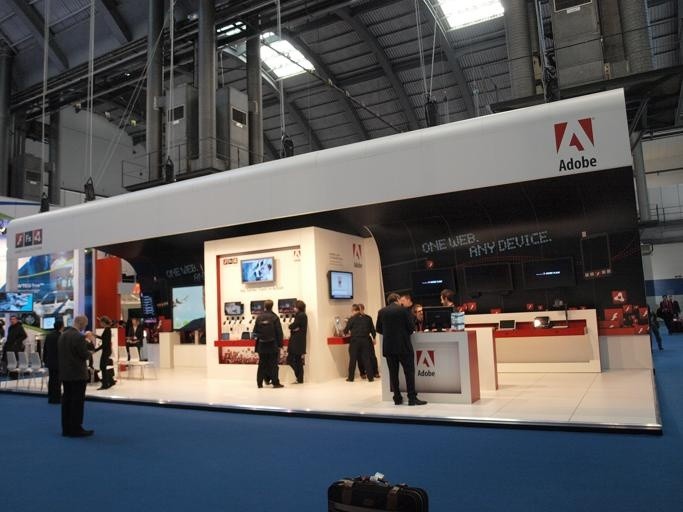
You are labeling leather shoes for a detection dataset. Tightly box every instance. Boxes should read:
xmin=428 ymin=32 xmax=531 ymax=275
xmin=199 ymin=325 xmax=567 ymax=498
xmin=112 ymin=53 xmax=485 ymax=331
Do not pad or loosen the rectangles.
xmin=394 ymin=398 xmax=403 ymax=405
xmin=69 ymin=427 xmax=94 ymax=437
xmin=408 ymin=397 xmax=427 ymax=405
xmin=273 ymin=384 xmax=284 ymax=389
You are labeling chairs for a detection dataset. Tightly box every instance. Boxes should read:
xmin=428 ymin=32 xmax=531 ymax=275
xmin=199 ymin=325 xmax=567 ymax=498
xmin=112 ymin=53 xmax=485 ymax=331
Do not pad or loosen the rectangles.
xmin=129 ymin=347 xmax=157 ymax=380
xmin=5 ymin=352 xmax=48 ymax=392
xmin=118 ymin=346 xmax=129 ymax=380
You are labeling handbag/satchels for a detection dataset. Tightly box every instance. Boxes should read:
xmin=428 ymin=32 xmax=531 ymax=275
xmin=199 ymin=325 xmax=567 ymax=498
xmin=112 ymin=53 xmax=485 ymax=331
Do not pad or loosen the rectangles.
xmin=327 ymin=471 xmax=429 ymax=512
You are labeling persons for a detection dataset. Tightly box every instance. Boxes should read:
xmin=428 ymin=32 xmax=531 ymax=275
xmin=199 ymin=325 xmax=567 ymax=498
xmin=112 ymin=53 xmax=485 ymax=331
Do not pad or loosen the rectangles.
xmin=410 ymin=304 xmax=425 ymax=331
xmin=343 ymin=304 xmax=374 ymax=382
xmin=376 ymin=293 xmax=427 ymax=406
xmin=358 ymin=304 xmax=380 ymax=378
xmin=57 ymin=315 xmax=94 ymax=436
xmin=441 ymin=289 xmax=457 ymax=312
xmin=82 ymin=317 xmax=143 ymax=390
xmin=644 ymin=304 xmax=664 ymax=350
xmin=401 ymin=295 xmax=414 ymax=310
xmin=253 ymin=261 xmax=272 ymax=281
xmin=251 ymin=300 xmax=284 ymax=388
xmin=288 ymin=300 xmax=307 ymax=384
xmin=657 ymin=294 xmax=680 ymax=336
xmin=43 ymin=321 xmax=62 ymax=404
xmin=2 ymin=316 xmax=27 ymax=368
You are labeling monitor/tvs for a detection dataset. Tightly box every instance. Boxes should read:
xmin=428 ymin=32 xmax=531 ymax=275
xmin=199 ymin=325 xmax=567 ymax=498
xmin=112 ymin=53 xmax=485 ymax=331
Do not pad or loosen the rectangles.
xmin=0 ymin=291 xmax=34 ymax=313
xmin=278 ymin=298 xmax=298 ymax=313
xmin=224 ymin=302 xmax=243 ymax=314
xmin=43 ymin=316 xmax=56 ymax=329
xmin=250 ymin=300 xmax=268 ymax=314
xmin=328 ymin=270 xmax=353 ymax=299
xmin=240 ymin=257 xmax=275 ymax=282
